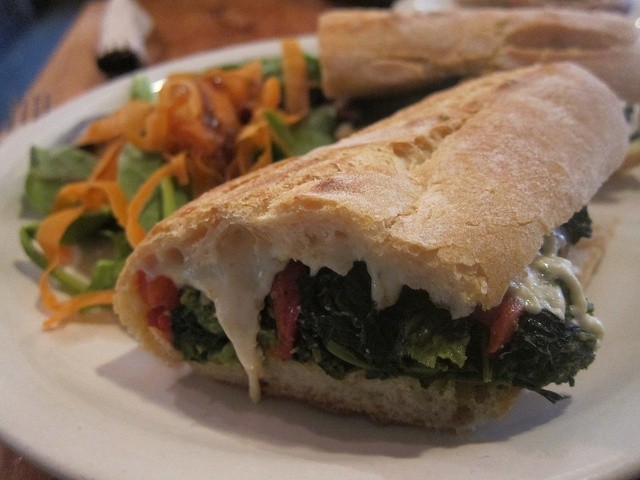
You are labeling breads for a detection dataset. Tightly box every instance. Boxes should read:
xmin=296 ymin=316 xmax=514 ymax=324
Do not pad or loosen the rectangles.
xmin=316 ymin=11 xmax=639 ymax=104
xmin=112 ymin=61 xmax=632 ymax=436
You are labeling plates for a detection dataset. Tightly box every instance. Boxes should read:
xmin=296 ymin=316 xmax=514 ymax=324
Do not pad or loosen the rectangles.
xmin=0 ymin=33 xmax=640 ymax=479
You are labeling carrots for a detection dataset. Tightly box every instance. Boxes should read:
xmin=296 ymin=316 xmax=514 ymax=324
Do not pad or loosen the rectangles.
xmin=38 ymin=40 xmax=306 ymax=332
